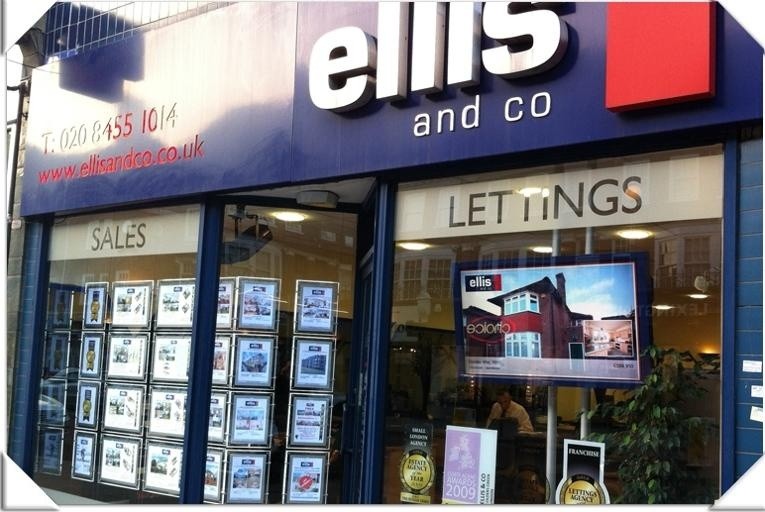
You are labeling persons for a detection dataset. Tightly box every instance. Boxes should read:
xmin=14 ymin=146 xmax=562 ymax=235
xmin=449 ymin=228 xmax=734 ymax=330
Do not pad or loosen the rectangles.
xmin=484 ymin=387 xmax=535 ymax=434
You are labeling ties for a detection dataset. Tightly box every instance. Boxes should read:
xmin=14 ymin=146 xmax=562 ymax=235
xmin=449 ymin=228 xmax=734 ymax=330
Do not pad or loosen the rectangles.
xmin=500 ymin=409 xmax=508 ymax=418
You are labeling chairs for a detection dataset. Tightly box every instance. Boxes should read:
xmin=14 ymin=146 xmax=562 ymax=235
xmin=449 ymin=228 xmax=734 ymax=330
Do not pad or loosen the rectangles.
xmin=508 ymin=428 xmax=562 ymax=504
xmin=486 ymin=414 xmax=520 ymax=477
